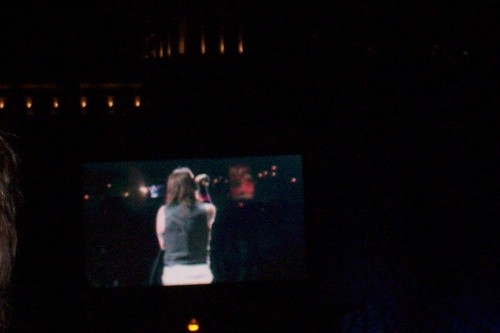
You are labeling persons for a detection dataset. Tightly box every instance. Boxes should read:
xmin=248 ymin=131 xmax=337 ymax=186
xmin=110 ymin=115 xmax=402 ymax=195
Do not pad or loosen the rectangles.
xmin=1 ymin=128 xmax=31 ymax=333
xmin=155 ymin=166 xmax=217 ymax=285
